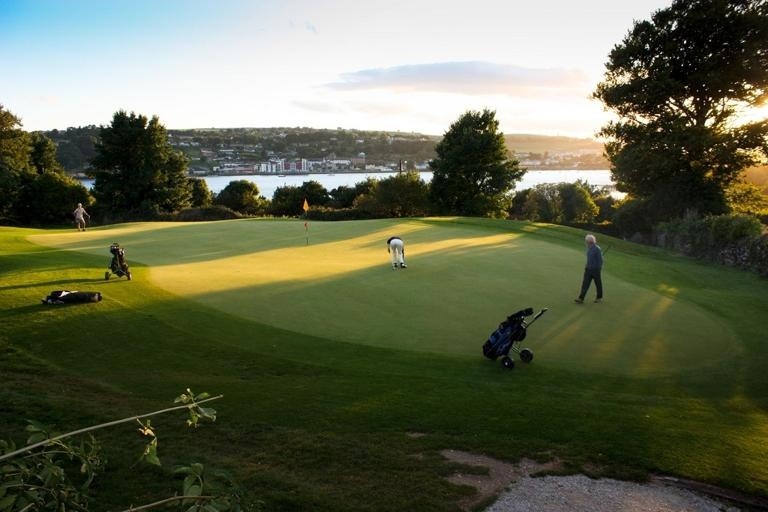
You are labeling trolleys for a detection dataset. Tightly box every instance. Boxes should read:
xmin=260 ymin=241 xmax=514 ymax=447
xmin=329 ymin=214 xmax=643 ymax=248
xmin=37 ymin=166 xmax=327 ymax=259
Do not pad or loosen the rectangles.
xmin=482 ymin=308 xmax=548 ymax=370
xmin=105 ymin=242 xmax=132 ymax=281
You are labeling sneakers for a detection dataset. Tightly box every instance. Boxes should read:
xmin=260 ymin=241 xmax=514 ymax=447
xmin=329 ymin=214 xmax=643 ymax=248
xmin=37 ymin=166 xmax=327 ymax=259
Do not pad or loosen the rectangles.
xmin=400 ymin=263 xmax=407 ymax=268
xmin=594 ymin=298 xmax=604 ymax=303
xmin=574 ymin=297 xmax=584 ymax=304
xmin=391 ymin=262 xmax=398 ymax=270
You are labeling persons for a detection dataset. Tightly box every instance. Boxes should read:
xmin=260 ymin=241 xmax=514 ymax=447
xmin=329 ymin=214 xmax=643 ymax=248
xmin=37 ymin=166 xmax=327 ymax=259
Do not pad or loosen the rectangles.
xmin=72 ymin=202 xmax=90 ymax=231
xmin=574 ymin=234 xmax=604 ymax=304
xmin=387 ymin=236 xmax=408 ymax=270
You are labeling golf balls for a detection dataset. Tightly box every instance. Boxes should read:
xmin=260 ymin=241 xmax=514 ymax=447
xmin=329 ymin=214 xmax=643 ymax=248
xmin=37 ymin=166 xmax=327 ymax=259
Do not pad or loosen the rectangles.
xmin=525 ymin=308 xmax=548 ymax=329
